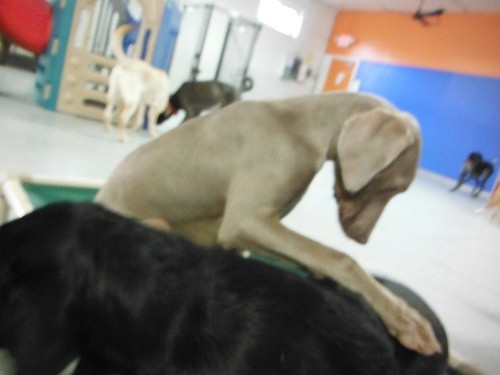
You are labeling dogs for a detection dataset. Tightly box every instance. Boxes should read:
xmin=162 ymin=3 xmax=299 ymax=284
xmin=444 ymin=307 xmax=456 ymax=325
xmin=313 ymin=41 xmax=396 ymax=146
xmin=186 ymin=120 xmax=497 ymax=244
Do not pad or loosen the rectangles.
xmin=1 ymin=200 xmax=467 ymax=375
xmin=449 ymin=152 xmax=497 ymax=199
xmin=94 ymin=90 xmax=444 ymax=358
xmin=477 ymin=177 xmax=500 ymax=223
xmin=155 ymin=80 xmax=242 ymax=126
xmin=103 ymin=23 xmax=183 ymax=142
